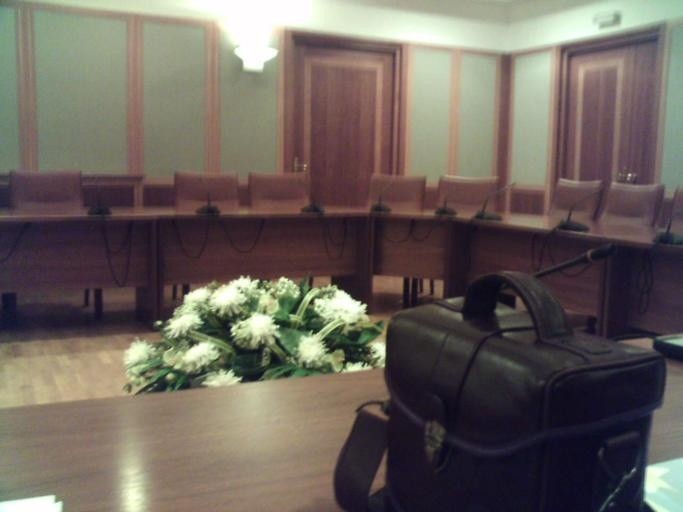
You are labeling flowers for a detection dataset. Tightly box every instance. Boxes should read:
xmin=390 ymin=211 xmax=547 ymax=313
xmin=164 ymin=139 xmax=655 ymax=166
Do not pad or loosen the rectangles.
xmin=125 ymin=276 xmax=384 ymax=392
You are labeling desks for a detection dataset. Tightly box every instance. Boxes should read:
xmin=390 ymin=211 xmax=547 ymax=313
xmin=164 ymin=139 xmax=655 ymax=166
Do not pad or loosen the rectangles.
xmin=0 ymin=203 xmax=683 ymax=334
xmin=1 ymin=325 xmax=682 ymax=512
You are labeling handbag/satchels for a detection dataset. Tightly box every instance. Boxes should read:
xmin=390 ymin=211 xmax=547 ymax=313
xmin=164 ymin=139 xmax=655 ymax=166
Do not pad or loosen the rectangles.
xmin=384 ymin=270 xmax=667 ymax=512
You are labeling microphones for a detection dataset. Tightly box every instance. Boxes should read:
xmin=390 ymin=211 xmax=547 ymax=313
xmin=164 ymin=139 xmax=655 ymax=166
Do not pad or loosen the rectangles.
xmin=474 ymin=182 xmax=516 ymax=220
xmin=196 ymin=173 xmax=221 ymax=213
xmin=533 ymin=242 xmax=616 ymax=278
xmin=370 ymin=181 xmax=395 ymax=211
xmin=558 ymin=187 xmax=606 ymax=232
xmin=301 ymin=191 xmax=325 ymax=212
xmin=653 ymin=184 xmax=683 ymax=244
xmin=434 ymin=185 xmax=458 ymax=214
xmin=87 ymin=175 xmax=112 ymax=215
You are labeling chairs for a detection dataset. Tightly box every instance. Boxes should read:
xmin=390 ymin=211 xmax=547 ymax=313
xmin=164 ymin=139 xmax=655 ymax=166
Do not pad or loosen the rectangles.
xmin=247 ymin=169 xmax=315 ymax=290
xmin=597 ymin=181 xmax=668 ymax=227
xmin=172 ymin=170 xmax=239 ymax=298
xmin=431 ymin=174 xmax=501 ymax=295
xmin=365 ymin=174 xmax=427 ymax=295
xmin=544 ymin=177 xmax=607 ymax=221
xmin=1 ymin=167 xmax=91 ymax=308
xmin=666 ymin=182 xmax=683 ymax=229
